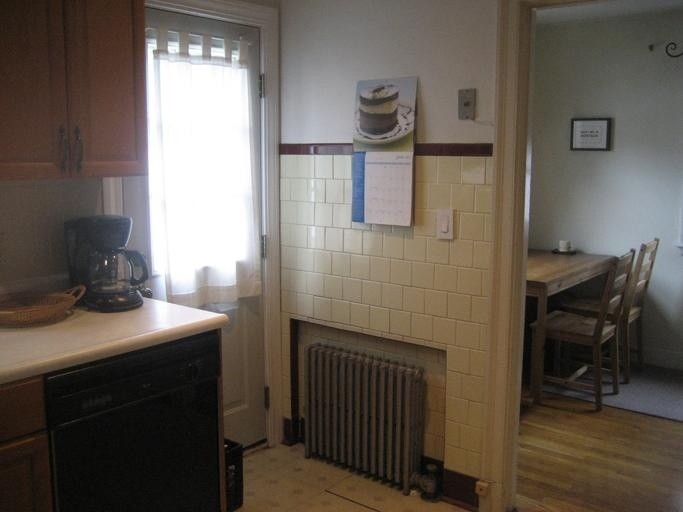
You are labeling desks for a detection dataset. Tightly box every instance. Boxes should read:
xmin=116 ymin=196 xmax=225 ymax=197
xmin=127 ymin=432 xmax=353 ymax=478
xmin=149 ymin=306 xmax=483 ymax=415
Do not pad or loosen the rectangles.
xmin=526 ymin=250 xmax=617 ymax=404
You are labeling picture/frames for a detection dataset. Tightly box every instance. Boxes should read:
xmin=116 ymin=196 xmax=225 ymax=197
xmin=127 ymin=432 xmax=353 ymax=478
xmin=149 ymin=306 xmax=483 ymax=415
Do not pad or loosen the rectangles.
xmin=570 ymin=118 xmax=611 ymax=151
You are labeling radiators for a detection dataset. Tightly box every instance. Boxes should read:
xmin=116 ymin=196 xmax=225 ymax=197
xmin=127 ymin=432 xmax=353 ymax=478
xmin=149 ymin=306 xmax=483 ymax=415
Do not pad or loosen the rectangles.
xmin=303 ymin=342 xmax=428 ymax=494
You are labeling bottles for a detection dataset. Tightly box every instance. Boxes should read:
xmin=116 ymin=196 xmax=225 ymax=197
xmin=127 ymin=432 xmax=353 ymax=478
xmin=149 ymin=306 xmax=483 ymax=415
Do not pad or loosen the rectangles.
xmin=424 ymin=465 xmax=439 ymax=497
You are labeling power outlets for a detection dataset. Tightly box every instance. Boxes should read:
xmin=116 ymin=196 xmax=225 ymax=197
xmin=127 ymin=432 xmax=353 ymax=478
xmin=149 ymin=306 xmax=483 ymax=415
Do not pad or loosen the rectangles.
xmin=458 ymin=88 xmax=476 ymax=120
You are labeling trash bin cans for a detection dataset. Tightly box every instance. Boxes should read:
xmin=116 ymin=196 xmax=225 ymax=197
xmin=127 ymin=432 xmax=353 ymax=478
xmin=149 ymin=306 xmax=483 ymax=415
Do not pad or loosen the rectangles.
xmin=224 ymin=437 xmax=243 ymax=512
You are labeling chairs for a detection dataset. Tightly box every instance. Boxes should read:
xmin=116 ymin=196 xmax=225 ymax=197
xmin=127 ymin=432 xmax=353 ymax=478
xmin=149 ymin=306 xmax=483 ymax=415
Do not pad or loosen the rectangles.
xmin=530 ymin=249 xmax=636 ymax=412
xmin=560 ymin=238 xmax=660 ymax=384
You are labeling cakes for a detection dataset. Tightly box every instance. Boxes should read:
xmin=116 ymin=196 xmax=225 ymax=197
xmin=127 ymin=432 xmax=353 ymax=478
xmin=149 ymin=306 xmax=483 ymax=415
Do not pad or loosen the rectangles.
xmin=359 ymin=83 xmax=400 ymax=136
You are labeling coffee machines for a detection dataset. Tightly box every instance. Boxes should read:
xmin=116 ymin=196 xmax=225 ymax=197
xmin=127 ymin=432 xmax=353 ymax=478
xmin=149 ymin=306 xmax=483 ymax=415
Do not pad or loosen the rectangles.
xmin=62 ymin=212 xmax=150 ymax=312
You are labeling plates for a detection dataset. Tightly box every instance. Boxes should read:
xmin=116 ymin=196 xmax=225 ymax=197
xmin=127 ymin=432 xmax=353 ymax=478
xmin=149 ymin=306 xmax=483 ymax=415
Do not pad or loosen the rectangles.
xmin=355 ymin=101 xmax=416 ymax=141
xmin=552 ymin=249 xmax=576 ymax=256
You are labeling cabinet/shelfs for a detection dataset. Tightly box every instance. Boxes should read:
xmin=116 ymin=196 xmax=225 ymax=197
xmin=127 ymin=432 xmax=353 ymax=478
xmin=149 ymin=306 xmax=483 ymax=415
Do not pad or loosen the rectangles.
xmin=0 ymin=0 xmax=146 ymax=179
xmin=0 ymin=375 xmax=53 ymax=512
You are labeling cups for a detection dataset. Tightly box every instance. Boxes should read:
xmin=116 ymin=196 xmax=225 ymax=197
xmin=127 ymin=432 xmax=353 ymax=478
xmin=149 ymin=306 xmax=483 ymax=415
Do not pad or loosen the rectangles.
xmin=558 ymin=240 xmax=570 ymax=251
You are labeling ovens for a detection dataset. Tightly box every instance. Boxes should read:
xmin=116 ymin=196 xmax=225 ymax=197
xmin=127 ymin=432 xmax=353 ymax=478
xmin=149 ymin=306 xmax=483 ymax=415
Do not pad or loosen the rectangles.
xmin=41 ymin=330 xmax=221 ymax=510
